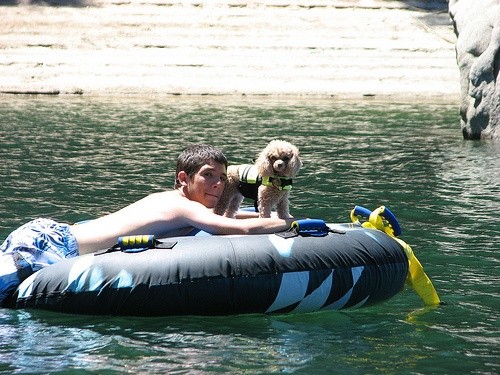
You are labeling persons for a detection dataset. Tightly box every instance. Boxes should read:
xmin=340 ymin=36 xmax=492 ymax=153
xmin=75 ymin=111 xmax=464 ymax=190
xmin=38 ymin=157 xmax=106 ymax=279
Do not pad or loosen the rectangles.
xmin=1 ymin=145 xmax=317 ymax=303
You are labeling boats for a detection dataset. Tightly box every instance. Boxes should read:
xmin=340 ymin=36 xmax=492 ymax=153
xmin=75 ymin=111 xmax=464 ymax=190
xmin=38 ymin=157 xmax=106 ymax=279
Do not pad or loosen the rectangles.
xmin=19 ymin=205 xmax=434 ymax=312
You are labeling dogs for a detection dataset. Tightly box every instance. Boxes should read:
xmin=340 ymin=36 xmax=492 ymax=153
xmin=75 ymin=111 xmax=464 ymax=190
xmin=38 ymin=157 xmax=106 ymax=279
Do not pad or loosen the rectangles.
xmin=213 ymin=140 xmax=304 ymax=219
